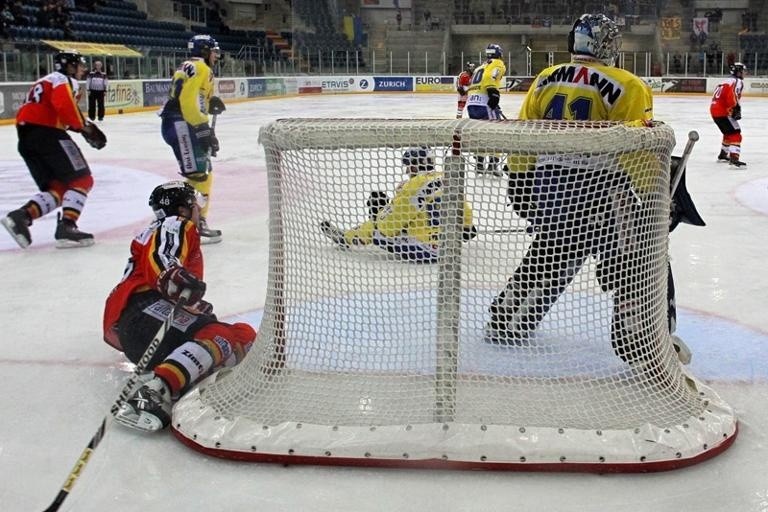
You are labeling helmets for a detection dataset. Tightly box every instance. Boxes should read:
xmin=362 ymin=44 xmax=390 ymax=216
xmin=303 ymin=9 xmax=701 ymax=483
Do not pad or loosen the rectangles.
xmin=53 ymin=51 xmax=89 ymax=76
xmin=402 ymin=145 xmax=435 ymax=172
xmin=148 ymin=179 xmax=207 ymax=220
xmin=486 ymin=43 xmax=503 ymax=59
xmin=567 ymin=13 xmax=623 ymax=66
xmin=728 ymin=61 xmax=747 ymax=79
xmin=186 ymin=34 xmax=221 ymax=59
xmin=464 ymin=61 xmax=479 ymax=74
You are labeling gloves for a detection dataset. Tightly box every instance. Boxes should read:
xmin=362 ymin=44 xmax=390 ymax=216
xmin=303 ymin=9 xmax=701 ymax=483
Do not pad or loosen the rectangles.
xmin=457 ymin=87 xmax=465 ymax=96
xmin=195 ymin=123 xmax=219 ymax=157
xmin=487 ymin=87 xmax=500 ymax=110
xmin=80 ymin=120 xmax=107 ymax=149
xmin=507 ymin=173 xmax=536 ymax=218
xmin=464 ymin=227 xmax=477 ymax=240
xmin=208 ymin=95 xmax=226 ymax=114
xmin=367 ymin=191 xmax=389 ymax=221
xmin=157 ymin=266 xmax=206 ymax=306
xmin=732 ymin=103 xmax=741 ymax=120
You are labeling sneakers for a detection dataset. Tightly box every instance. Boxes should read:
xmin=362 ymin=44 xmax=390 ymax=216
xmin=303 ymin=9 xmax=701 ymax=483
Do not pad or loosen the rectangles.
xmin=475 ymin=156 xmax=486 ymax=174
xmin=113 ymin=373 xmax=173 ymax=432
xmin=728 ymin=158 xmax=746 ymax=168
xmin=670 ymin=337 xmax=693 ymax=363
xmin=487 ymin=157 xmax=503 ymax=177
xmin=320 ymin=221 xmax=350 ymax=251
xmin=483 ymin=320 xmax=532 ymax=345
xmin=718 ymin=150 xmax=730 ymax=161
xmin=54 ymin=212 xmax=95 ymax=248
xmin=196 ymin=217 xmax=223 ymax=244
xmin=1 ymin=208 xmax=31 ymax=248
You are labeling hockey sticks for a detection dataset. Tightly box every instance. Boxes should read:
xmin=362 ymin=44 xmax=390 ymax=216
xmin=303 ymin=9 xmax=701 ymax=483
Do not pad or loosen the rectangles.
xmin=475 ymin=227 xmax=537 ymax=237
xmin=179 ymin=107 xmax=218 ymax=181
xmin=499 ymin=79 xmax=517 ymax=90
xmin=44 ymin=286 xmax=190 ymax=512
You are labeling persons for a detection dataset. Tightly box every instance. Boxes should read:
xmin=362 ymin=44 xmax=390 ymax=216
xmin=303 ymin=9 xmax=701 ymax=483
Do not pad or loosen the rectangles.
xmin=420 ymin=2 xmax=440 ymax=30
xmin=162 ymin=60 xmax=175 ymax=79
xmin=0 ymin=48 xmax=108 ymax=250
xmin=652 ymin=8 xmax=767 ymax=78
xmin=0 ymin=1 xmax=131 ymax=80
xmin=710 ymin=62 xmax=749 ymax=169
xmin=395 ymin=9 xmax=405 ymax=31
xmin=451 ymin=62 xmax=478 ymax=159
xmin=484 ymin=14 xmax=694 ymax=387
xmin=158 ymin=33 xmax=223 ymax=243
xmin=466 ymin=43 xmax=504 ymax=177
xmin=102 ymin=181 xmax=257 ymax=425
xmin=85 ymin=60 xmax=109 ymax=122
xmin=320 ymin=149 xmax=479 ymax=262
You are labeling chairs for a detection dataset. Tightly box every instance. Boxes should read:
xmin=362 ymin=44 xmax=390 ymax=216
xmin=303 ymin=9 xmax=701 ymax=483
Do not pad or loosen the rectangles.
xmin=0 ymin=0 xmax=284 ymax=62
